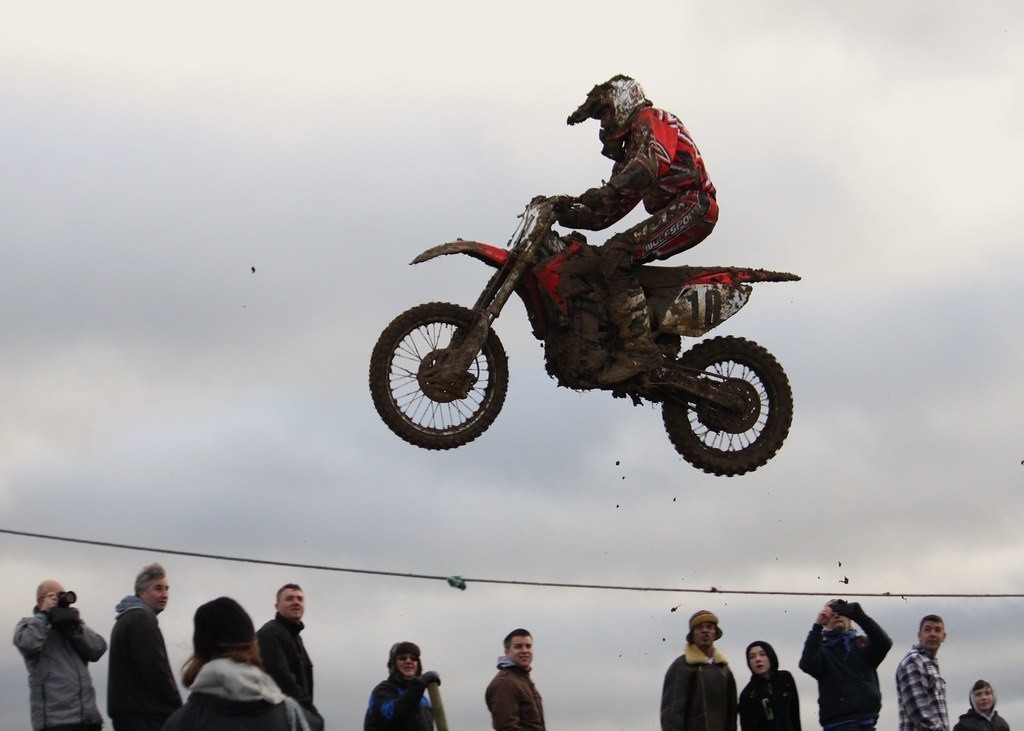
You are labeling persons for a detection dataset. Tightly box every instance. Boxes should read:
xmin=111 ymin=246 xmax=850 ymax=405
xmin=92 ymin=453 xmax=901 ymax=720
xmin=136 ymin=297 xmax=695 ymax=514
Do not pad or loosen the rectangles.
xmin=363 ymin=641 xmax=441 ymax=731
xmin=529 ymin=74 xmax=719 ymax=387
xmin=739 ymin=641 xmax=802 ymax=731
xmin=660 ymin=610 xmax=738 ymax=731
xmin=256 ymin=583 xmax=325 ymax=731
xmin=895 ymin=614 xmax=950 ymax=731
xmin=163 ymin=596 xmax=311 ymax=731
xmin=106 ymin=563 xmax=184 ymax=731
xmin=798 ymin=599 xmax=894 ymax=731
xmin=13 ymin=579 xmax=108 ymax=731
xmin=952 ymin=679 xmax=1010 ymax=731
xmin=485 ymin=629 xmax=547 ymax=731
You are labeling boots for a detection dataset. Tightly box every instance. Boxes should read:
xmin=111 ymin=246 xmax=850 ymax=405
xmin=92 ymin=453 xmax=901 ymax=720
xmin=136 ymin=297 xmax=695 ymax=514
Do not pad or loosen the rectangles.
xmin=598 ymin=286 xmax=664 ymax=385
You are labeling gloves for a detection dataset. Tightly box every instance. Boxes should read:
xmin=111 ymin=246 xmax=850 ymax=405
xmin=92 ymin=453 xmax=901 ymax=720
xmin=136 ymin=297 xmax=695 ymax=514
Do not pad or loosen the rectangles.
xmin=418 ymin=671 xmax=441 ymax=687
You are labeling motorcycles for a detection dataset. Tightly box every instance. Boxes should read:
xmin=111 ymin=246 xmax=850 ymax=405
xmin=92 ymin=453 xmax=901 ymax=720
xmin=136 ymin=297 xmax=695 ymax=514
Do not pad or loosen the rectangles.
xmin=369 ymin=195 xmax=801 ymax=478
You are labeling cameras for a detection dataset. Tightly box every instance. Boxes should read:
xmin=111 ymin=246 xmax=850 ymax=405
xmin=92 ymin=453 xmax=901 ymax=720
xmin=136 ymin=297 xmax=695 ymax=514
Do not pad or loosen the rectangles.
xmin=55 ymin=591 xmax=77 ymax=608
xmin=831 ymin=599 xmax=848 ymax=613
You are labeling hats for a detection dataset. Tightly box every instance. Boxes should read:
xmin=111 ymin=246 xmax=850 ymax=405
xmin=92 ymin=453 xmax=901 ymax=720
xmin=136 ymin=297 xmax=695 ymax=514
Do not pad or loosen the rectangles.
xmin=686 ymin=610 xmax=723 ymax=643
xmin=387 ymin=641 xmax=422 ymax=673
xmin=192 ymin=596 xmax=256 ymax=656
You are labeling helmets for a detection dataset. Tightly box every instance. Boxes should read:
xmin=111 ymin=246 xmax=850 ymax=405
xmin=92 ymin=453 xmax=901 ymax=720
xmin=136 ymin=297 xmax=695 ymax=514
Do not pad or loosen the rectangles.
xmin=566 ymin=74 xmax=653 ymax=162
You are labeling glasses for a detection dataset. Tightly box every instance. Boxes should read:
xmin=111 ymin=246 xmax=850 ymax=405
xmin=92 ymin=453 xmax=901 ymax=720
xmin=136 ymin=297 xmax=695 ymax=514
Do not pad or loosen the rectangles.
xmin=396 ymin=654 xmax=418 ymax=662
